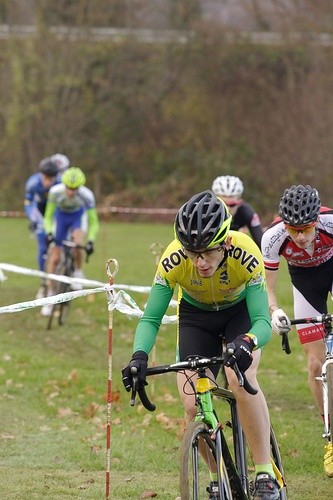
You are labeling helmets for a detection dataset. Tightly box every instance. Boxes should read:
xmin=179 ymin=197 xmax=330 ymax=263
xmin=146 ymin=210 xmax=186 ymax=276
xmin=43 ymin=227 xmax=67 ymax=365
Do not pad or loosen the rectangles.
xmin=51 ymin=154 xmax=69 ymax=170
xmin=175 ymin=190 xmax=232 ymax=248
xmin=39 ymin=159 xmax=59 ymax=175
xmin=212 ymin=175 xmax=244 ymax=196
xmin=279 ymin=185 xmax=321 ymax=226
xmin=63 ymin=167 xmax=85 ymax=188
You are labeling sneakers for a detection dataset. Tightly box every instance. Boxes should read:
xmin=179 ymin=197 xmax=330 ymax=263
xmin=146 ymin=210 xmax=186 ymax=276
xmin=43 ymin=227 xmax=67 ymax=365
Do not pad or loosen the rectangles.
xmin=252 ymin=472 xmax=280 ymax=500
xmin=206 ymin=481 xmax=219 ymax=500
xmin=322 ymin=443 xmax=333 ymax=477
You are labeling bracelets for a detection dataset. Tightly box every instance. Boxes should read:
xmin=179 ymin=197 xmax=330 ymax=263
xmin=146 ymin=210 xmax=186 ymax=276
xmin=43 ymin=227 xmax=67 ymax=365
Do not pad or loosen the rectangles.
xmin=269 ymin=303 xmax=277 ymax=308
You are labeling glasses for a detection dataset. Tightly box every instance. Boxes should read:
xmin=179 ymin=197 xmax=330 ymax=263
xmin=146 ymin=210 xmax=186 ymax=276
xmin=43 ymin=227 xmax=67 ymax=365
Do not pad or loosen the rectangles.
xmin=284 ymin=218 xmax=318 ymax=237
xmin=183 ymin=242 xmax=226 ymax=260
xmin=225 ymin=202 xmax=239 ymax=207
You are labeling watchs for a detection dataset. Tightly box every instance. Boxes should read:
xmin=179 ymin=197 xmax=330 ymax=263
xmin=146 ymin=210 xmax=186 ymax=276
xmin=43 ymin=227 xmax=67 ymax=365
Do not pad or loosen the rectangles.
xmin=245 ymin=333 xmax=258 ymax=351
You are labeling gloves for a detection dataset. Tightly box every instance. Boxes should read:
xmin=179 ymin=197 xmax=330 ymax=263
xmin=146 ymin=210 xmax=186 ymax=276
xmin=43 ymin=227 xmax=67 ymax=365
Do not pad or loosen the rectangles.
xmin=122 ymin=350 xmax=149 ymax=391
xmin=84 ymin=241 xmax=93 ymax=254
xmin=46 ymin=233 xmax=55 ymax=246
xmin=271 ymin=309 xmax=291 ymax=335
xmin=224 ymin=334 xmax=257 ymax=373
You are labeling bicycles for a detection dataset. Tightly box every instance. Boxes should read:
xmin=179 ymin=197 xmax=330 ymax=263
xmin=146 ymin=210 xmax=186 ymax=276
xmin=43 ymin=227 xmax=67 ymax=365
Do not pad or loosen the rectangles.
xmin=279 ymin=313 xmax=333 ymax=462
xmin=128 ymin=342 xmax=287 ymax=500
xmin=27 ymin=223 xmax=95 ymax=331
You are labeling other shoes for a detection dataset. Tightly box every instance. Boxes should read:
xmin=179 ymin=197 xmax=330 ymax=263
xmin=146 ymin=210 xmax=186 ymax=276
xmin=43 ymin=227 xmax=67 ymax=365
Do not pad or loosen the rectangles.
xmin=35 ymin=285 xmax=45 ymax=300
xmin=71 ymin=272 xmax=84 ymax=291
xmin=42 ymin=296 xmax=54 ymax=317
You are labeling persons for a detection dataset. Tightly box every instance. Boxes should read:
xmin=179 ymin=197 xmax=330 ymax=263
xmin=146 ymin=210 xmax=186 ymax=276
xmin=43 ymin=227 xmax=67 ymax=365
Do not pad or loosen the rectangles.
xmin=121 ymin=189 xmax=280 ymax=500
xmin=261 ymin=185 xmax=333 ymax=477
xmin=23 ymin=153 xmax=100 ymax=316
xmin=212 ymin=175 xmax=264 ymax=252
